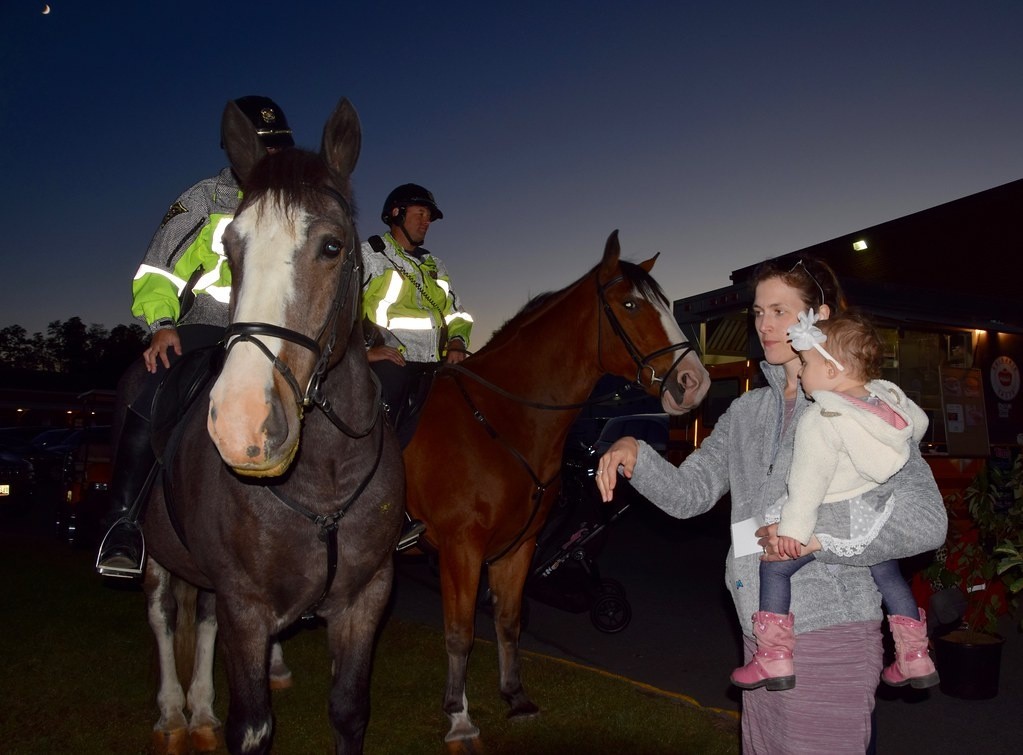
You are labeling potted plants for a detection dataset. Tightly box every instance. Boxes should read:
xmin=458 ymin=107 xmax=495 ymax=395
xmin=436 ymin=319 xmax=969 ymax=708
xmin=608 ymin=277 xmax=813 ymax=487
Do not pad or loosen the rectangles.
xmin=919 ymin=452 xmax=1022 ymax=700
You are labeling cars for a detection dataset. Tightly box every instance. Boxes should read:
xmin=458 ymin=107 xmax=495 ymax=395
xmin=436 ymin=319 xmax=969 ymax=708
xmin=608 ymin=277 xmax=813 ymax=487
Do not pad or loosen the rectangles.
xmin=589 ymin=412 xmax=689 ymax=463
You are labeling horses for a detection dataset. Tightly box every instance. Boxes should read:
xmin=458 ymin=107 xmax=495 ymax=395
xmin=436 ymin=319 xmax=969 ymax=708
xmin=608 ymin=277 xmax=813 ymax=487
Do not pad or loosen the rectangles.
xmin=109 ymin=92 xmax=408 ymax=755
xmin=387 ymin=229 xmax=710 ymax=755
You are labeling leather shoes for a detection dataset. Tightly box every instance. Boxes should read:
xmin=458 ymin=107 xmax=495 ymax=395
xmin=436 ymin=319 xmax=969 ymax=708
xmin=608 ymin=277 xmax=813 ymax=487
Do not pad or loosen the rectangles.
xmin=399 ymin=520 xmax=427 ymax=540
xmin=98 ymin=531 xmax=141 ymax=571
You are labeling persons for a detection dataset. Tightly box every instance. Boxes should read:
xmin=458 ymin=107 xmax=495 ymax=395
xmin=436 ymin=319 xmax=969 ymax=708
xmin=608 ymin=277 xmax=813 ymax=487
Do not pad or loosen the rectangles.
xmin=360 ymin=183 xmax=474 ymax=539
xmin=596 ymin=255 xmax=948 ymax=755
xmin=106 ymin=95 xmax=295 ymax=566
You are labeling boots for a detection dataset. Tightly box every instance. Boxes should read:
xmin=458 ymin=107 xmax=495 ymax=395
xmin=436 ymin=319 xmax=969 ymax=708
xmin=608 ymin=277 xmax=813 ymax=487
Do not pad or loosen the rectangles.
xmin=878 ymin=605 xmax=938 ymax=690
xmin=731 ymin=609 xmax=797 ymax=690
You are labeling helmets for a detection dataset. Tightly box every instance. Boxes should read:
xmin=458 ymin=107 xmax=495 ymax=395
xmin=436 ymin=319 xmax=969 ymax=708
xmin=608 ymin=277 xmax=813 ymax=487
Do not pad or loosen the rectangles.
xmin=220 ymin=95 xmax=296 ymax=149
xmin=381 ymin=183 xmax=444 ymax=227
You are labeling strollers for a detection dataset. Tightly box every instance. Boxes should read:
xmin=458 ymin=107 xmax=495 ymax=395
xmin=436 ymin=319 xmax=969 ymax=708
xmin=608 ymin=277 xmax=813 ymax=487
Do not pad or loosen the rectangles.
xmin=480 ymin=438 xmax=695 ymax=637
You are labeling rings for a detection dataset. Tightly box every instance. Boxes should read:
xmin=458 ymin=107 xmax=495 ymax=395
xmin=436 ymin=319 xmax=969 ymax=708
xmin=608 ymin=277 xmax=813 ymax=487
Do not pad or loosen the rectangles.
xmin=763 ymin=546 xmax=767 ymax=554
xmin=597 ymin=470 xmax=603 ymax=475
